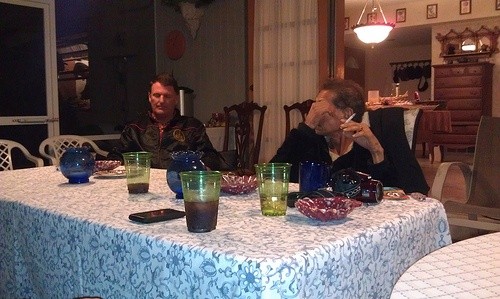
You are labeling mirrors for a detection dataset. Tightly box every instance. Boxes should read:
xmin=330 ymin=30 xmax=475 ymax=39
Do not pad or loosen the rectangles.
xmin=439 ymin=26 xmax=496 ymax=57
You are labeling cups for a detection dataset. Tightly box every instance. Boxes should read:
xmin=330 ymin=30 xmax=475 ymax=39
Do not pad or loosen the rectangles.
xmin=368 ymin=90 xmax=379 ymax=104
xmin=180 ymin=169 xmax=222 ymax=234
xmin=254 ymin=163 xmax=293 ymax=215
xmin=300 ymin=160 xmax=327 ymax=191
xmin=122 ymin=152 xmax=152 ymax=193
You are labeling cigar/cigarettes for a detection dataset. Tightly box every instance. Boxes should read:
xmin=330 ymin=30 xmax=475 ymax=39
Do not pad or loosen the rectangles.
xmin=346 ymin=113 xmax=355 ymax=125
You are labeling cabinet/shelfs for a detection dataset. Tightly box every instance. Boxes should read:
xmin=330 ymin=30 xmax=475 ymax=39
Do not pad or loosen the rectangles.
xmin=432 ymin=63 xmax=494 ymax=152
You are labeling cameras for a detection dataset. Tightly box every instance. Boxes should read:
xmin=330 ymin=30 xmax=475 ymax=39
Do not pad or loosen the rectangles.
xmin=330 ymin=167 xmax=384 ymax=204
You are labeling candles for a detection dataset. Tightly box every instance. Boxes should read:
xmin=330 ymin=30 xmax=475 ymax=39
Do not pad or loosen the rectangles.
xmin=396 ymin=83 xmax=400 ymax=97
xmin=179 ymin=90 xmax=184 ymax=115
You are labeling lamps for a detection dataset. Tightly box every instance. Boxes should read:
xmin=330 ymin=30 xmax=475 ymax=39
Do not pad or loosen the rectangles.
xmin=351 ymin=0 xmax=395 ymax=49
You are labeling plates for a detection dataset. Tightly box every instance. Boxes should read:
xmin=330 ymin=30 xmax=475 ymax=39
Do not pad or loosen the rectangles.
xmin=95 ymin=170 xmax=142 ymax=177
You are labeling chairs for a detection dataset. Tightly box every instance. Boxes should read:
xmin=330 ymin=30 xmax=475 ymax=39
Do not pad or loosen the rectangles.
xmin=368 ymin=108 xmax=429 ymax=196
xmin=431 ymin=115 xmax=500 ymax=236
xmin=284 ymin=99 xmax=316 ymax=139
xmin=360 ymin=91 xmax=440 ymax=149
xmin=223 ymin=102 xmax=267 ymax=176
xmin=0 ymin=139 xmax=44 ymax=171
xmin=39 ymin=134 xmax=109 ymax=166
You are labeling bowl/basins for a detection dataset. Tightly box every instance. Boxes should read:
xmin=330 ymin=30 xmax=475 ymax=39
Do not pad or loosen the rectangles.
xmin=220 ymin=174 xmax=259 ymax=194
xmin=95 ymin=161 xmax=122 ymax=169
xmin=295 ymin=196 xmax=354 ymax=221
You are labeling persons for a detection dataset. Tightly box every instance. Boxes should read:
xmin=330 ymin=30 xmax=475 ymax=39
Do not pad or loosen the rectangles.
xmin=107 ymin=73 xmax=219 ymax=172
xmin=265 ymin=78 xmax=402 ymax=186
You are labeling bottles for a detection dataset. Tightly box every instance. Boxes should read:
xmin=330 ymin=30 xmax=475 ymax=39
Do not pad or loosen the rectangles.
xmin=166 ymin=151 xmax=207 ymax=198
xmin=59 ymin=146 xmax=95 ymax=183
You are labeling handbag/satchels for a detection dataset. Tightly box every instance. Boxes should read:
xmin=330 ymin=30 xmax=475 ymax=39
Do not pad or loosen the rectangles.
xmin=211 ymin=149 xmax=238 ymax=171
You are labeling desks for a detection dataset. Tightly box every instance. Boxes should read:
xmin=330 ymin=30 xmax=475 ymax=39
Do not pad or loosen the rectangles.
xmin=369 ymin=105 xmax=453 ymax=164
xmin=0 ymin=166 xmax=452 ymax=299
xmin=205 ymin=127 xmax=241 ymax=155
xmin=388 ymin=232 xmax=500 ymax=299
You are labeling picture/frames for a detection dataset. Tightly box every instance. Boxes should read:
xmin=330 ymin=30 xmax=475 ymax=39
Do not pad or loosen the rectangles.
xmin=344 ymin=0 xmax=500 ymax=30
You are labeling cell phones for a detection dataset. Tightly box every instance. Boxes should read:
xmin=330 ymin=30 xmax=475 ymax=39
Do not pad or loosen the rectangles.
xmin=128 ymin=208 xmax=185 ymax=223
